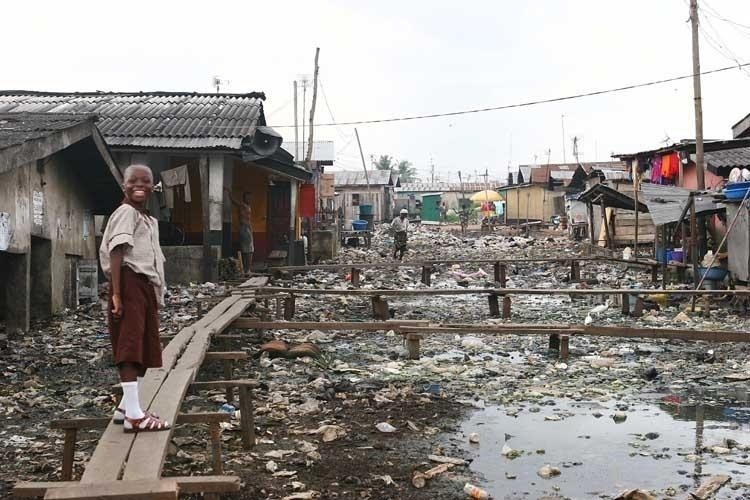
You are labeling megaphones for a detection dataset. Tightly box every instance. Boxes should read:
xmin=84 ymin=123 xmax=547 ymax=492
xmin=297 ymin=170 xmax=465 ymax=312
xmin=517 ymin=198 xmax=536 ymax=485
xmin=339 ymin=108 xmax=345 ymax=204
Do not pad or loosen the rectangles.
xmin=252 ymin=128 xmax=280 ymax=156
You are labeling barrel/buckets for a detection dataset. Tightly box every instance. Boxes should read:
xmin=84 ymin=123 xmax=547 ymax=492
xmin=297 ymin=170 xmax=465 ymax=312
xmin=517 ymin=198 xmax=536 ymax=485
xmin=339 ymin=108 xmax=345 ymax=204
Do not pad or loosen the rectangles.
xmin=353 ymin=219 xmax=368 ymax=230
xmin=344 ymin=219 xmax=353 ymax=230
xmin=359 ymin=204 xmax=371 ymax=213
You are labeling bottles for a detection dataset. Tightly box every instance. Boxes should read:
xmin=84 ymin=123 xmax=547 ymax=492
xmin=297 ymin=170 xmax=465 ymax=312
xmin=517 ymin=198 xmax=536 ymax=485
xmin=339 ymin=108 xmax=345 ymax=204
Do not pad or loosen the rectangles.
xmin=221 ymin=402 xmax=234 ymax=413
xmin=464 ymin=482 xmax=490 ymax=500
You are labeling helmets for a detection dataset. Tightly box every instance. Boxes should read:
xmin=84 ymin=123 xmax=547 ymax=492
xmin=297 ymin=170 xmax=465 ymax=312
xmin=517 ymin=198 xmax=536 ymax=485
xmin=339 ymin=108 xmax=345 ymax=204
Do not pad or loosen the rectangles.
xmin=400 ymin=209 xmax=408 ymax=214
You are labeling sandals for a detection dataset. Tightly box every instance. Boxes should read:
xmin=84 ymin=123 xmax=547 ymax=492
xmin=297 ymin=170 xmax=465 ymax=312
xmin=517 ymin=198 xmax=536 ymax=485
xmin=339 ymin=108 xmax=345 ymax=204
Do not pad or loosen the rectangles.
xmin=113 ymin=408 xmax=172 ymax=433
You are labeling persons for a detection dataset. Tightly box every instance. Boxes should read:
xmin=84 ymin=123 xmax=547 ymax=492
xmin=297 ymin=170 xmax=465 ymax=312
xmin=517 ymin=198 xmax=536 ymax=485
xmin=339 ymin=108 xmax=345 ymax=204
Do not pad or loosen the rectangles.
xmin=98 ymin=164 xmax=172 ymax=432
xmin=391 ymin=201 xmax=498 ymax=260
xmin=229 ymin=192 xmax=254 ymax=273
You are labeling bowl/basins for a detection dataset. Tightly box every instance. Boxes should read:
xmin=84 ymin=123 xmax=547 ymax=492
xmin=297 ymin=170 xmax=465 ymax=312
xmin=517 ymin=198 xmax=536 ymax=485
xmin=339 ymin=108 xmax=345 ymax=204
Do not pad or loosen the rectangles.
xmin=671 ymin=251 xmax=688 ymax=262
xmin=351 ymin=220 xmax=368 ymax=230
xmin=697 ymin=267 xmax=728 ymax=281
xmin=723 ymin=181 xmax=750 ymax=199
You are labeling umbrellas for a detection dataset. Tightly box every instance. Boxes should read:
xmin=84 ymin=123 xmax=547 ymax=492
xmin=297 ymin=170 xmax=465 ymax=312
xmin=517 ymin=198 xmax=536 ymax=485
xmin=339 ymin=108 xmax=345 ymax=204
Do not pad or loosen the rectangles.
xmin=470 ymin=189 xmax=506 ymax=230
